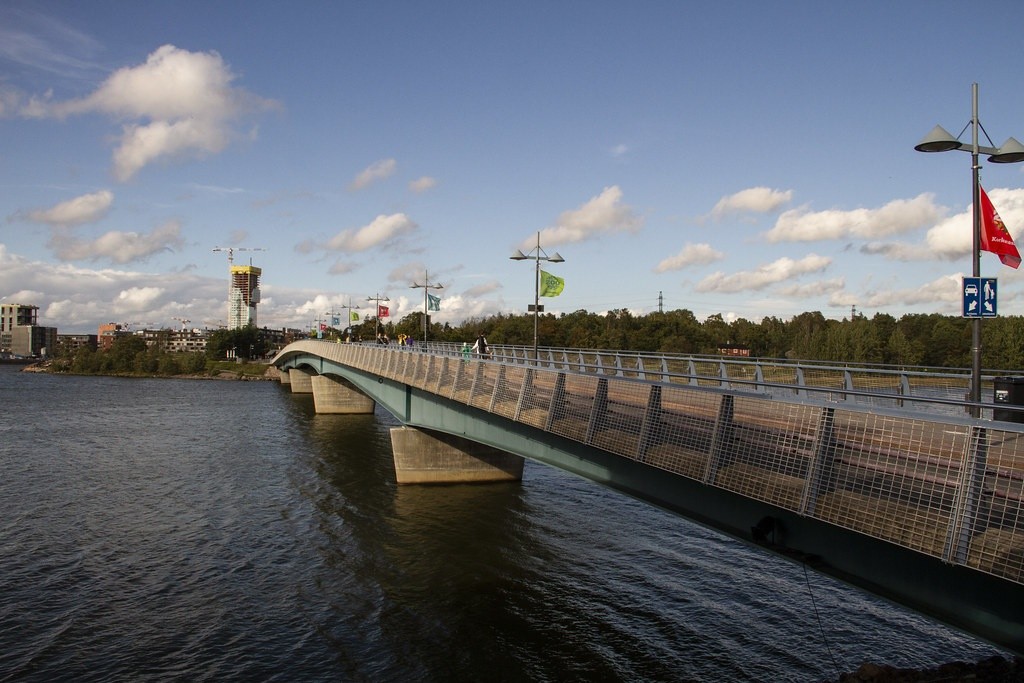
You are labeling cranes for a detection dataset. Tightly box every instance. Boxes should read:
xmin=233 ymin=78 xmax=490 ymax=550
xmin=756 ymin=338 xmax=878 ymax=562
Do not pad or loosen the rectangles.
xmin=170 ymin=316 xmax=191 ymax=330
xmin=213 ymin=246 xmax=271 ymax=266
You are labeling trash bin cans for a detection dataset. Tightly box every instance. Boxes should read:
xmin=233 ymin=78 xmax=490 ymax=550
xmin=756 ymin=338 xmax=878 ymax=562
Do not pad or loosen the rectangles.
xmin=992 ymin=375 xmax=1024 ymax=433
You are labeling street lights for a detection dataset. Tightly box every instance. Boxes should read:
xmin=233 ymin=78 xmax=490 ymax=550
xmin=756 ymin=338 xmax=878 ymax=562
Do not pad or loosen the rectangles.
xmin=914 ymin=84 xmax=1024 ymax=534
xmin=366 ymin=293 xmax=390 ymax=345
xmin=410 ymin=269 xmax=443 ymax=353
xmin=341 ymin=298 xmax=361 ymax=343
xmin=509 ymin=231 xmax=565 ymax=377
xmin=325 ymin=307 xmax=342 ymax=341
xmin=315 ymin=314 xmax=326 ymax=339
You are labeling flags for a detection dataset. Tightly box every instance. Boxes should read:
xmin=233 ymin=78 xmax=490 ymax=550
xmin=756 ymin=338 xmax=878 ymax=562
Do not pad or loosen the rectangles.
xmin=379 ymin=306 xmax=389 ymax=317
xmin=351 ymin=312 xmax=359 ymax=321
xmin=539 ymin=270 xmax=564 ymax=297
xmin=428 ymin=294 xmax=440 ymax=311
xmin=333 ymin=318 xmax=340 ymax=325
xmin=978 ymin=183 xmax=1021 ymax=269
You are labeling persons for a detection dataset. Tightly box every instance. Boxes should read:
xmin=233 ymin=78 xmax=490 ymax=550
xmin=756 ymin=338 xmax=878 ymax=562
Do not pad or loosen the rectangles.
xmin=460 ymin=342 xmax=469 ymax=352
xmin=398 ymin=334 xmax=407 ymax=346
xmin=471 ymin=334 xmax=489 ymax=359
xmin=377 ymin=333 xmax=389 ymax=344
xmin=358 ymin=335 xmax=363 ymax=346
xmin=406 ymin=334 xmax=414 ymax=346
xmin=351 ymin=336 xmax=356 ymax=342
